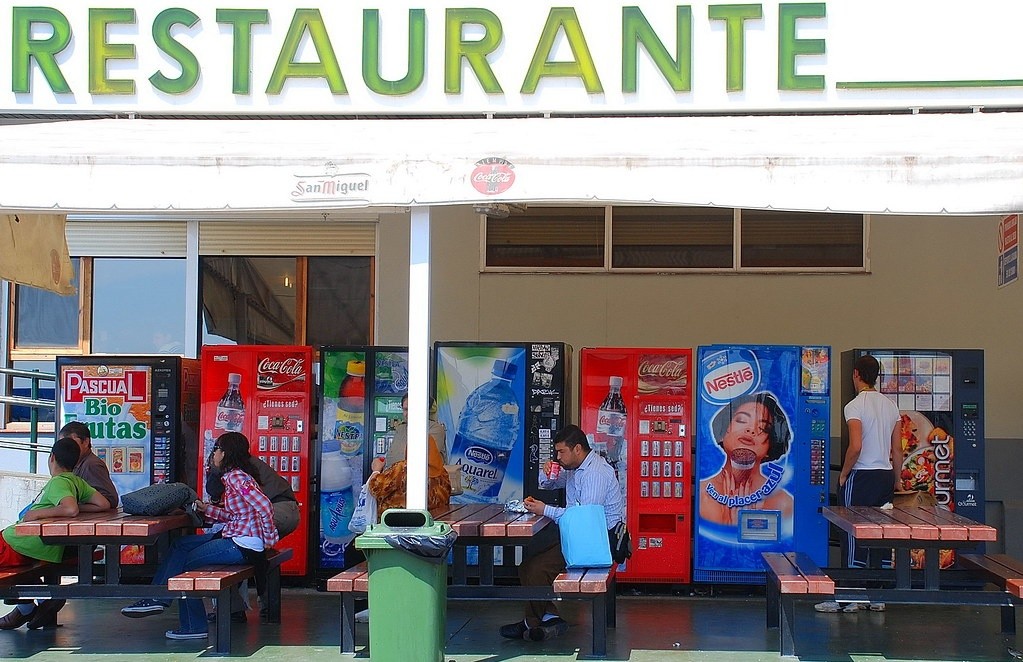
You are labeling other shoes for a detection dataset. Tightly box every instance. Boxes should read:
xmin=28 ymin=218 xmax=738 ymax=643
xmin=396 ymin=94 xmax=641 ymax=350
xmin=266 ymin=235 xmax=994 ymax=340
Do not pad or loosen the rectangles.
xmin=120 ymin=599 xmax=164 ymax=618
xmin=354 ymin=609 xmax=368 ymax=622
xmin=207 ymin=610 xmax=248 ymax=623
xmin=165 ymin=625 xmax=209 ymax=639
xmin=27 ymin=599 xmax=67 ymax=628
xmin=0 ymin=604 xmax=37 ymax=630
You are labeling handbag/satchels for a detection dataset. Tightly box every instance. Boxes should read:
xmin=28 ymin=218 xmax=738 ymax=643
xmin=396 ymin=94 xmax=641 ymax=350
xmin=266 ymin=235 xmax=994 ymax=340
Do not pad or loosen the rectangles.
xmin=19 ymin=502 xmax=32 ymax=519
xmin=558 ymin=505 xmax=613 ymax=568
xmin=443 ymin=464 xmax=463 ymax=496
xmin=120 ymin=482 xmax=204 ymax=527
xmin=347 ymin=471 xmax=381 ymax=534
xmin=611 ymin=521 xmax=632 ymax=564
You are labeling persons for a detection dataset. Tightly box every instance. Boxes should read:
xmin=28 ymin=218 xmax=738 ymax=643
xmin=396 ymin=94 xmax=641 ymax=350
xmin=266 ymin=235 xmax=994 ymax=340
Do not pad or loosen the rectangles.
xmin=0 ymin=422 xmax=119 ymax=630
xmin=344 ymin=434 xmax=451 ymax=572
xmin=0 ymin=438 xmax=110 ymax=569
xmin=206 ymin=456 xmax=300 ymax=622
xmin=386 ymin=393 xmax=447 ymax=469
xmin=814 ymin=355 xmax=903 ymax=613
xmin=498 ymin=425 xmax=627 ymax=641
xmin=700 ymin=393 xmax=794 ymax=526
xmin=120 ymin=432 xmax=278 ymax=639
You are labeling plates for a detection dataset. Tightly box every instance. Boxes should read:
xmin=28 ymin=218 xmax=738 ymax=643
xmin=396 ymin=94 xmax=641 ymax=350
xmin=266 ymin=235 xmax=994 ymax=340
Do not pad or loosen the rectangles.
xmin=901 ymin=446 xmax=936 ymax=470
xmin=898 ymin=410 xmax=934 ymax=462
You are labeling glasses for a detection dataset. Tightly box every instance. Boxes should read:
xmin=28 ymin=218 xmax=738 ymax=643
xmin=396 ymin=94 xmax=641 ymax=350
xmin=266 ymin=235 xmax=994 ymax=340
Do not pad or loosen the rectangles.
xmin=213 ymin=442 xmax=224 ymax=452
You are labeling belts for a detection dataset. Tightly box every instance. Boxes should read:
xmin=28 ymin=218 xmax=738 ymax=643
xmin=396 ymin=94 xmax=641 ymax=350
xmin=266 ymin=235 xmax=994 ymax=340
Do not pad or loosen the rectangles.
xmin=270 ymin=496 xmax=300 ymax=505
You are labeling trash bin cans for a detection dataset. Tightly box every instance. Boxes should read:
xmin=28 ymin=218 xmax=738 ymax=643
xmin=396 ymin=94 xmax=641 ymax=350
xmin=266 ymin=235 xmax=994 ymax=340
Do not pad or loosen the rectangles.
xmin=354 ymin=509 xmax=458 ymax=662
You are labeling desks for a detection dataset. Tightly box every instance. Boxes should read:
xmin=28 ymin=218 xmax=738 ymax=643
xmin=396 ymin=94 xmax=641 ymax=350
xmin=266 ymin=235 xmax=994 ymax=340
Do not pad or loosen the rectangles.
xmin=425 ymin=500 xmax=558 ymax=601
xmin=819 ymin=504 xmax=1009 ymax=609
xmin=13 ymin=503 xmax=202 ymax=599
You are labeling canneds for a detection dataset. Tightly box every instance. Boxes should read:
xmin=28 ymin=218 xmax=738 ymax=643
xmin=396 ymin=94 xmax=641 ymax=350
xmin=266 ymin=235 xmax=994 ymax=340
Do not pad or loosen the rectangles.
xmin=547 ymin=462 xmax=559 ymax=480
xmin=641 ymin=440 xmax=683 ymax=498
xmin=258 ymin=436 xmax=302 ymax=492
xmin=376 ymin=437 xmax=393 ymax=454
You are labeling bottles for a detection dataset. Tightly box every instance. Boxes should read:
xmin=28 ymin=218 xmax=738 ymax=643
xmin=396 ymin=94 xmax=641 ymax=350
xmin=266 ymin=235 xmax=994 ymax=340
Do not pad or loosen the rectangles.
xmin=207 ymin=373 xmax=245 ymax=458
xmin=321 ymin=440 xmax=357 ymax=544
xmin=334 ymin=360 xmax=365 ymax=511
xmin=203 ymin=430 xmax=214 ymax=503
xmin=595 ymin=376 xmax=628 ymax=482
xmin=447 ymin=360 xmax=520 ymax=564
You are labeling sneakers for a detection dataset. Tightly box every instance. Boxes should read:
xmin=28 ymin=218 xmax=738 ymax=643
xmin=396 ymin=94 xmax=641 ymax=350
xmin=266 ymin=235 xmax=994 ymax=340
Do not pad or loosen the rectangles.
xmin=523 ymin=616 xmax=569 ymax=642
xmin=814 ymin=602 xmax=858 ymax=612
xmin=500 ymin=617 xmax=543 ymax=640
xmin=859 ymin=602 xmax=885 ymax=612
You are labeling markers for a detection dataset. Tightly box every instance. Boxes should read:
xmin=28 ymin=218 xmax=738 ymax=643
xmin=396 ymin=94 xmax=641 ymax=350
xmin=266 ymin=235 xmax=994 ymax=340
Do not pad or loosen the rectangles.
xmin=193 ymin=503 xmax=197 ymax=512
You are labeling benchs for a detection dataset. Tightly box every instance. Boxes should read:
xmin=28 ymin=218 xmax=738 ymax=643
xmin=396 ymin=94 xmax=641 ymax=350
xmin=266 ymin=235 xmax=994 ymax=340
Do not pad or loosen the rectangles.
xmin=0 ymin=546 xmax=104 ymax=628
xmin=554 ymin=560 xmax=618 ymax=660
xmin=326 ymin=558 xmax=369 ymax=654
xmin=166 ymin=547 xmax=294 ymax=656
xmin=760 ymin=551 xmax=835 ymax=659
xmin=958 ymin=551 xmax=1023 ymax=635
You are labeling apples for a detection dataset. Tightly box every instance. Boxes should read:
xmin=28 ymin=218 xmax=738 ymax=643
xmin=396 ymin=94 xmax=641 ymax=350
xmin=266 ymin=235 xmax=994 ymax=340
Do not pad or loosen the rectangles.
xmin=120 ymin=544 xmax=144 ymax=564
